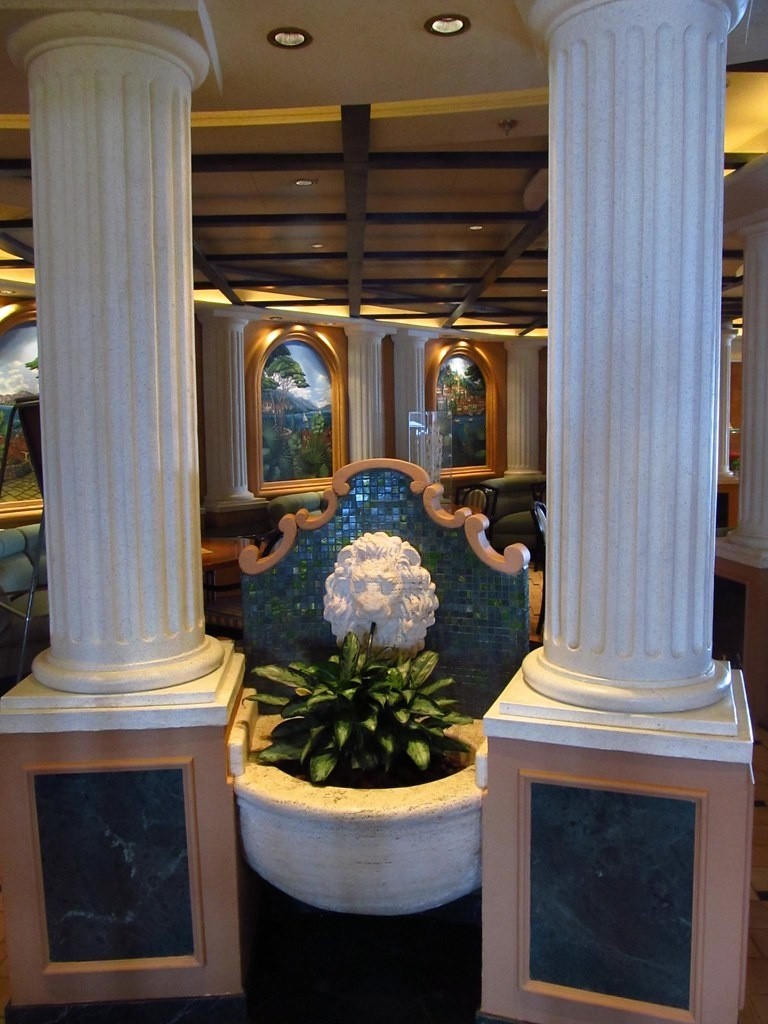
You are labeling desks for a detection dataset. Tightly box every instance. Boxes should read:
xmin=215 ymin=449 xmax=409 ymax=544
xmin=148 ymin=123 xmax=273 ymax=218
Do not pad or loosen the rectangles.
xmin=201 ymin=537 xmax=257 ymax=607
xmin=441 ymin=502 xmax=481 ymax=517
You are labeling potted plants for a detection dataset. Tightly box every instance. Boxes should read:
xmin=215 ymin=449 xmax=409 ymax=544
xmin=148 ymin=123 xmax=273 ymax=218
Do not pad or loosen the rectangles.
xmin=231 ymin=632 xmax=489 ymax=917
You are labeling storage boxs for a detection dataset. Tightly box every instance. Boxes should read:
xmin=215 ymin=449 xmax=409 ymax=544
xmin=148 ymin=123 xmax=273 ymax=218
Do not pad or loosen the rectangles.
xmin=408 ymin=411 xmax=453 ymax=502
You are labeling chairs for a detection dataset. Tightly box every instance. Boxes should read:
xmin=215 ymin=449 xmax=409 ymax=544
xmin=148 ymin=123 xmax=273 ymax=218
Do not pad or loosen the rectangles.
xmin=259 ymin=531 xmax=286 ymax=559
xmin=455 ymin=483 xmax=500 ymax=544
xmin=531 ymin=501 xmax=548 ymax=636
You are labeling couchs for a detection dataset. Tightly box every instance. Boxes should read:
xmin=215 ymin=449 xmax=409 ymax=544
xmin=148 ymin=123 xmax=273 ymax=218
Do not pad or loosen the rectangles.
xmin=267 ymin=492 xmax=323 ymax=534
xmin=19 ymin=522 xmax=47 ymax=586
xmin=0 ymin=527 xmax=50 ymax=679
xmin=479 ymin=474 xmax=547 ymax=550
